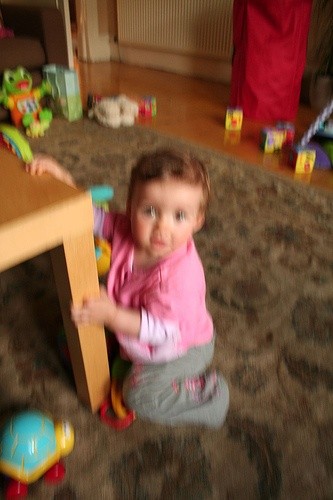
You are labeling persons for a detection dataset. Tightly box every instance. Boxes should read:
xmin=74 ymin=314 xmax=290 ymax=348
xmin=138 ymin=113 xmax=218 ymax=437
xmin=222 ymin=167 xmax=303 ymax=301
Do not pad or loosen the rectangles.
xmin=25 ymin=146 xmax=229 ymax=432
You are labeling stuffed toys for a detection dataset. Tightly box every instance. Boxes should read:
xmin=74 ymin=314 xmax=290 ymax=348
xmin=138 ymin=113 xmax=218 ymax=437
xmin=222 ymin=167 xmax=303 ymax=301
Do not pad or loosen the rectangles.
xmin=0 ymin=67 xmax=52 ymax=140
xmin=88 ymin=94 xmax=138 ymax=129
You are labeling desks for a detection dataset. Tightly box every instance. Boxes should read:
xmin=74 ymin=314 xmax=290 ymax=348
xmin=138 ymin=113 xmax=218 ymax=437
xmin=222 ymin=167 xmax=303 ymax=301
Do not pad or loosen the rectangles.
xmin=0 ymin=142 xmax=112 ymax=417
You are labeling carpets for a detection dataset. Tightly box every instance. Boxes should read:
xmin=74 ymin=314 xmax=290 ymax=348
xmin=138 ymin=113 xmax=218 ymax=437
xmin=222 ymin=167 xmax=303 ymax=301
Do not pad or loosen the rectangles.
xmin=1 ymin=95 xmax=333 ymax=500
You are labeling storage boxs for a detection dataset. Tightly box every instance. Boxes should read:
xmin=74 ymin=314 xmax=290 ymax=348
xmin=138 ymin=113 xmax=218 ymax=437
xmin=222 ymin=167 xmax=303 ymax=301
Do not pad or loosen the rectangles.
xmin=43 ymin=64 xmax=83 ymax=122
